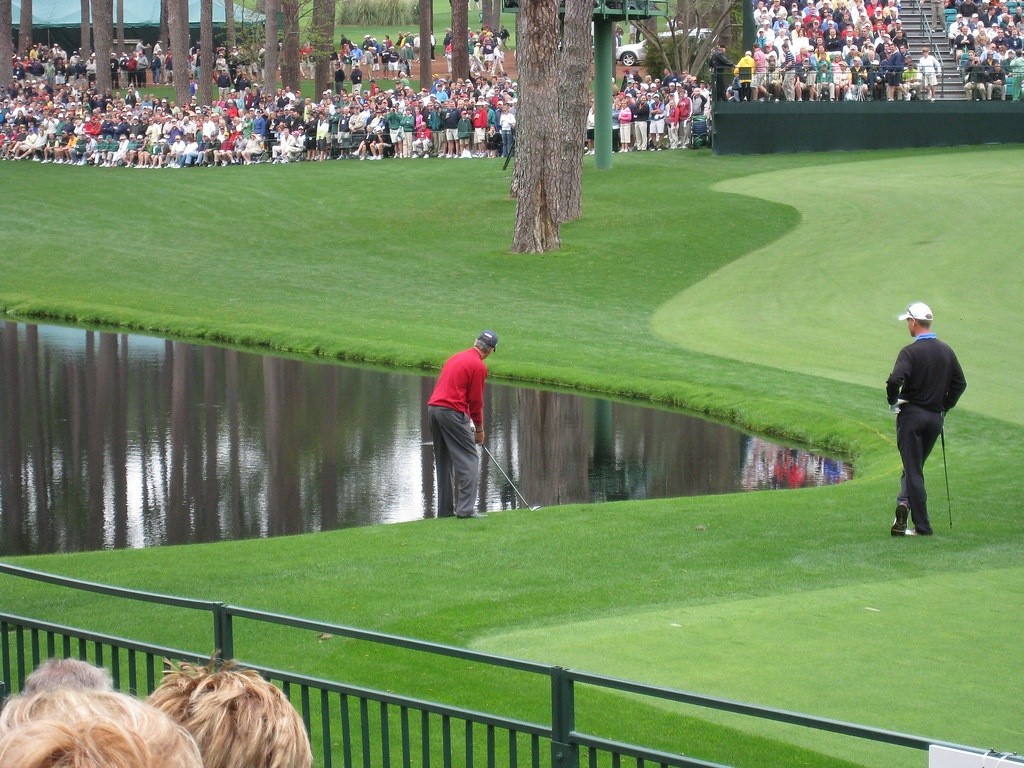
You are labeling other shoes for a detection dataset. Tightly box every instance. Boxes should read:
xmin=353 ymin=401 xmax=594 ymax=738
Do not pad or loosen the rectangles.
xmin=891 ymin=503 xmax=908 ymax=536
xmin=906 ymin=528 xmax=922 ymax=536
xmin=266 ymin=150 xmax=498 ymax=165
xmin=457 ymin=512 xmax=488 ymax=519
xmin=0 ymin=154 xmax=252 ymax=169
xmin=618 ymin=144 xmax=688 ymax=154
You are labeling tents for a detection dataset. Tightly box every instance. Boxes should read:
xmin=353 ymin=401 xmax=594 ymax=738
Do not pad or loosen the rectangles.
xmin=11 ymin=0 xmax=269 ymax=62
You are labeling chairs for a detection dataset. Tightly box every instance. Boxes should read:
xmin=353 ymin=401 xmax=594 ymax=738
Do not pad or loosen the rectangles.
xmin=252 ymin=140 xmax=270 ymax=163
xmin=944 ymin=0 xmax=1024 ymax=96
xmin=331 ymin=137 xmax=362 ymax=159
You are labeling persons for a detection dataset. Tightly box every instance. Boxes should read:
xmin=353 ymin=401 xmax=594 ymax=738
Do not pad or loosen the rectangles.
xmin=0 ymin=0 xmax=1024 ymax=168
xmin=887 ymin=302 xmax=967 ymax=536
xmin=427 ymin=332 xmax=497 ymax=521
xmin=0 ymin=651 xmax=312 ymax=768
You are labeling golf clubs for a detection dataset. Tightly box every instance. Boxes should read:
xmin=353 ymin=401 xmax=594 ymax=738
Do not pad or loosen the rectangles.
xmin=482 ymin=443 xmax=540 ymax=512
xmin=941 ymin=427 xmax=952 ymax=527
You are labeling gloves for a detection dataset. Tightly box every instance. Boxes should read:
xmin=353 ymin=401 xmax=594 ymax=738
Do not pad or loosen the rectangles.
xmin=889 ymin=399 xmax=909 ymax=414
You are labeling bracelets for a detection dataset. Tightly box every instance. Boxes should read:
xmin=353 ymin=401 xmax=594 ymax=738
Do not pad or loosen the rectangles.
xmin=476 ymin=428 xmax=484 ymax=433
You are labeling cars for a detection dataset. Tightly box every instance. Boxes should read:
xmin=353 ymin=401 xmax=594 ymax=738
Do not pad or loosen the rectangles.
xmin=615 ymin=27 xmax=714 ymax=67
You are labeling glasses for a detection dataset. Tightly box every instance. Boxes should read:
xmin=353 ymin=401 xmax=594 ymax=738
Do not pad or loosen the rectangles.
xmin=906 ymin=305 xmax=916 ymax=321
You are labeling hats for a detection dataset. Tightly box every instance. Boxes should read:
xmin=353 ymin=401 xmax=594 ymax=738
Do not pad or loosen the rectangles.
xmin=0 ymin=27 xmax=711 ymax=143
xmin=898 ymin=303 xmax=933 ymax=321
xmin=477 ymin=331 xmax=498 ymax=353
xmin=752 ymin=0 xmax=1024 ymax=62
